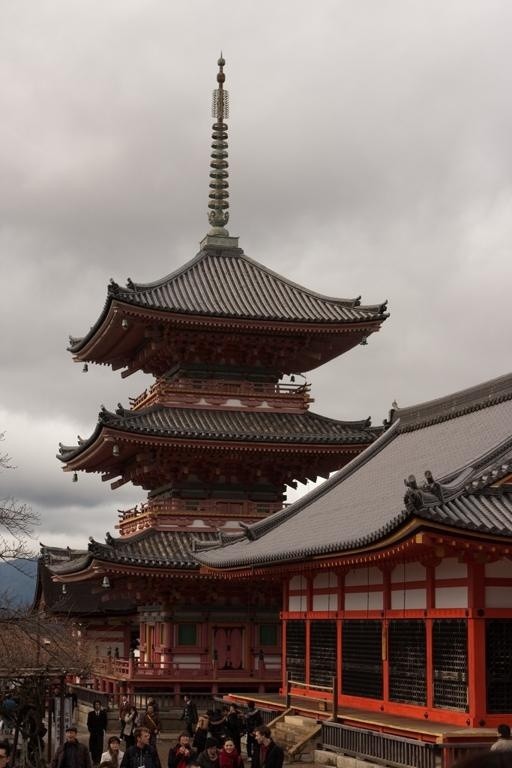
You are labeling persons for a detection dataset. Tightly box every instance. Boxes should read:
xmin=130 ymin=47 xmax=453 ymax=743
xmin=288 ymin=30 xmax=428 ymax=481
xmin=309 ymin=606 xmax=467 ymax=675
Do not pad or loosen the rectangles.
xmin=487 ymin=724 xmax=511 ymax=753
xmin=1 ymin=684 xmax=285 ymax=768
